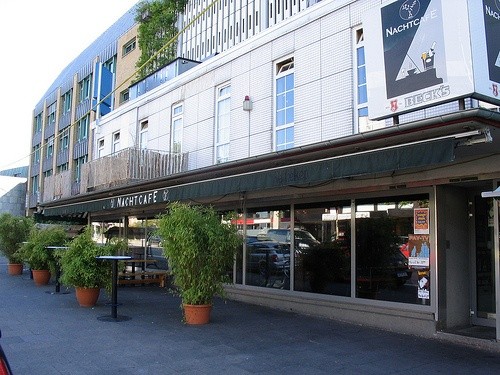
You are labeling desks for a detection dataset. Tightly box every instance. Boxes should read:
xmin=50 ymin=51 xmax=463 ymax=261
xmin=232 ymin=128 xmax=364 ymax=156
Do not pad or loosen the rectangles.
xmin=22 ymin=242 xmax=34 ymax=280
xmin=45 ymin=246 xmax=72 ymax=294
xmin=94 ymin=256 xmax=132 ymax=322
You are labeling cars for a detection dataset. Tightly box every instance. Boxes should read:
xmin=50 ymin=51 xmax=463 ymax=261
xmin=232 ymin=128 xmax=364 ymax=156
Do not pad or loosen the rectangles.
xmin=233 ymin=227 xmax=412 ymax=292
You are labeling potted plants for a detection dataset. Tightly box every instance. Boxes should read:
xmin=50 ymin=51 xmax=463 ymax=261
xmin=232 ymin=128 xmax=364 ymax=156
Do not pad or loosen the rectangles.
xmin=10 ymin=224 xmax=77 ymax=286
xmin=146 ymin=199 xmax=247 ymax=325
xmin=0 ymin=212 xmax=37 ymax=276
xmin=51 ymin=224 xmax=135 ymax=308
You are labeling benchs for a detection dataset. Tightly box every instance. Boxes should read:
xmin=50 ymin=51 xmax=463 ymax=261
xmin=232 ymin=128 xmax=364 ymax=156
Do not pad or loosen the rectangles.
xmin=120 ymin=260 xmax=156 ymax=273
